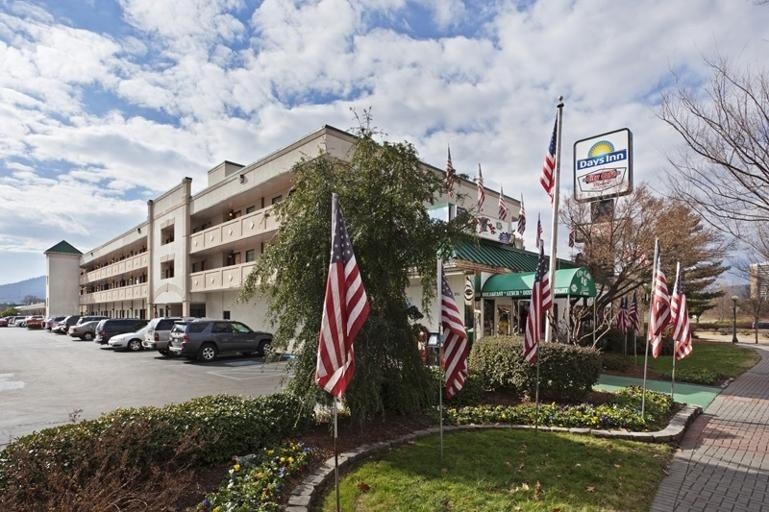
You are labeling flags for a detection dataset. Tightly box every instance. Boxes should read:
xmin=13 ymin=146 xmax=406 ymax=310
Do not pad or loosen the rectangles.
xmin=647 ymin=250 xmax=671 ymax=359
xmin=516 ymin=198 xmax=526 ymax=235
xmin=538 ymin=109 xmax=558 ymax=203
xmin=436 ymin=259 xmax=470 ymax=400
xmin=477 ymin=165 xmax=485 ymax=211
xmin=568 ymin=220 xmax=575 ymax=248
xmin=522 ymin=245 xmax=553 ymax=367
xmin=498 ymin=187 xmax=508 ymax=221
xmin=314 ymin=199 xmax=371 ymax=400
xmin=536 ymin=218 xmax=543 ymax=248
xmin=616 ymin=293 xmax=642 ymax=337
xmin=670 ymin=267 xmax=693 ymax=361
xmin=445 ymin=147 xmax=456 ymax=200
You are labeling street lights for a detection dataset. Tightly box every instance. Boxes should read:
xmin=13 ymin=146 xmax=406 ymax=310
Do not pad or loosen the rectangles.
xmin=732 ymin=296 xmax=739 ymax=342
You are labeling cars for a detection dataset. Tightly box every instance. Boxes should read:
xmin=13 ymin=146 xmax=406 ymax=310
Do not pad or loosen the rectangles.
xmin=0 ymin=315 xmax=274 ymax=362
